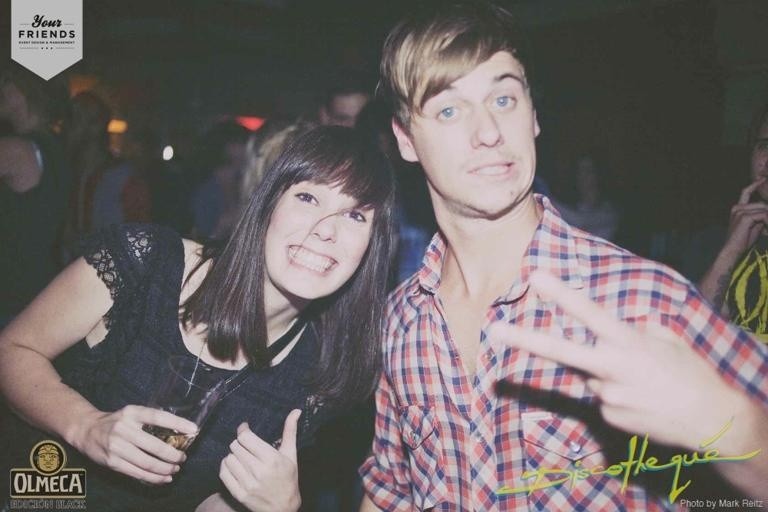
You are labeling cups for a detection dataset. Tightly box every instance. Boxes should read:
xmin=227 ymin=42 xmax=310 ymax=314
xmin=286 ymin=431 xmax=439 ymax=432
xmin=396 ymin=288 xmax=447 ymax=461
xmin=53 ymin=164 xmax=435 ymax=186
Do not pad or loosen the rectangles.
xmin=130 ymin=354 xmax=228 ymax=489
